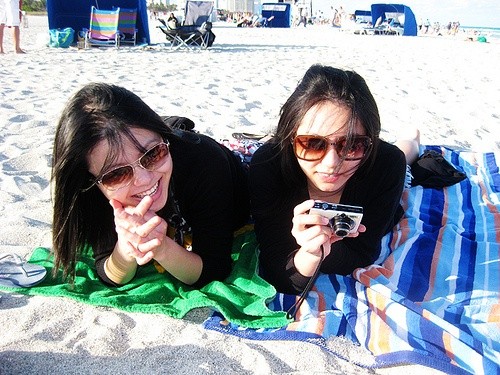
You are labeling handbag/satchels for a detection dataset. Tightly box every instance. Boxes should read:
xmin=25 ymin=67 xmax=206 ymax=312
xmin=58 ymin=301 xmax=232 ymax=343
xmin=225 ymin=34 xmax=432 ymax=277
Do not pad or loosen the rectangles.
xmin=219 ymin=132 xmax=275 ymax=162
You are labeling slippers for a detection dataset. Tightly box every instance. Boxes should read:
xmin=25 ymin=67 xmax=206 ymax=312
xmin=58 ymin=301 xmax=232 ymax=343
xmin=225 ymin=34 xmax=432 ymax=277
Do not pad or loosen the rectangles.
xmin=0 ymin=252 xmax=47 ymax=287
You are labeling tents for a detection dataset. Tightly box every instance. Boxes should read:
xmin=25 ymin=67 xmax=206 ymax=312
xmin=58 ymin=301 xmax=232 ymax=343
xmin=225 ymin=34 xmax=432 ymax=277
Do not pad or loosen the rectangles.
xmin=47 ymin=1 xmax=151 ymax=47
xmin=370 ymin=4 xmax=417 ymax=37
xmin=261 ymin=3 xmax=292 ymax=28
xmin=354 ymin=9 xmax=373 ymax=29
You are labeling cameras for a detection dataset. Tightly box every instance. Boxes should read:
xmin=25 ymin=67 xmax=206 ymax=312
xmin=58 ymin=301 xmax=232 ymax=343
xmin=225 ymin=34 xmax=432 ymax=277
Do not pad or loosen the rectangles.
xmin=304 ymin=199 xmax=363 ymax=237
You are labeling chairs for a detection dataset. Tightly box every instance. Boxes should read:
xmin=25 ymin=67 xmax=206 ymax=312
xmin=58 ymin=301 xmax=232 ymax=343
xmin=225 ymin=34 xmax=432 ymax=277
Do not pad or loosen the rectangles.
xmin=108 ymin=6 xmax=138 ymax=47
xmin=85 ymin=6 xmax=120 ymax=50
xmin=156 ymin=15 xmax=209 ymax=53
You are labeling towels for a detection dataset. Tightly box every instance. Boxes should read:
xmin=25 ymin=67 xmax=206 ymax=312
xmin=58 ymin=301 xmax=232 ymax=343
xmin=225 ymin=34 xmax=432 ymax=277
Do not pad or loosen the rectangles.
xmin=1 ymin=145 xmax=500 ymax=375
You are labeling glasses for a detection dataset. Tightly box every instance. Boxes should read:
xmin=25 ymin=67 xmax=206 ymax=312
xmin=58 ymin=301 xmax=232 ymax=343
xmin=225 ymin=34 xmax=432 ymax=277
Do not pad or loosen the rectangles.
xmin=288 ymin=133 xmax=372 ymax=161
xmin=90 ymin=137 xmax=170 ymax=191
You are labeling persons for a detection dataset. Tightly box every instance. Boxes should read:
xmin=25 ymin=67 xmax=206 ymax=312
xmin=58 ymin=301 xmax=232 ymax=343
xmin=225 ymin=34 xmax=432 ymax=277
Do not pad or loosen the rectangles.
xmin=0 ymin=0 xmax=28 ymax=55
xmin=50 ymin=83 xmax=276 ymax=289
xmin=247 ymin=62 xmax=421 ymax=295
xmin=146 ymin=0 xmax=489 ymax=48
